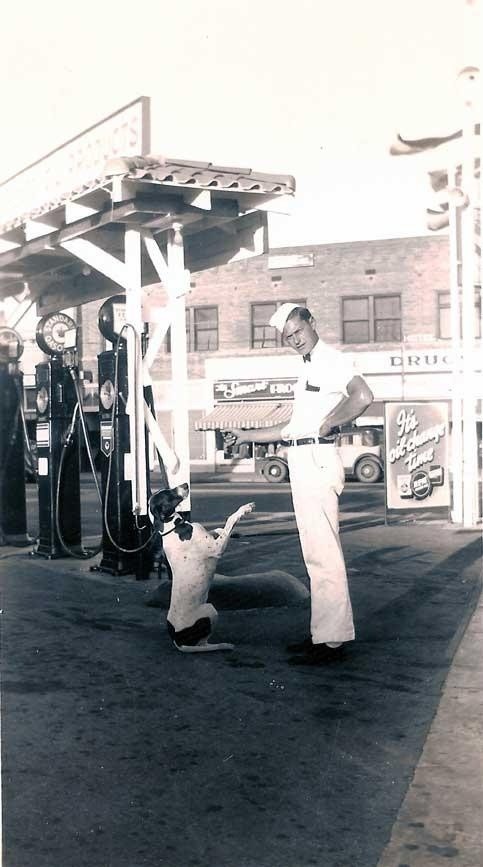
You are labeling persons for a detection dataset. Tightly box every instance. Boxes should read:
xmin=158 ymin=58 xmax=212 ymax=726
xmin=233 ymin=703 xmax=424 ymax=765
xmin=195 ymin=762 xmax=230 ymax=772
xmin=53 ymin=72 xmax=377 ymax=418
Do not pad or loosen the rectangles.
xmin=218 ymin=298 xmax=374 ymax=670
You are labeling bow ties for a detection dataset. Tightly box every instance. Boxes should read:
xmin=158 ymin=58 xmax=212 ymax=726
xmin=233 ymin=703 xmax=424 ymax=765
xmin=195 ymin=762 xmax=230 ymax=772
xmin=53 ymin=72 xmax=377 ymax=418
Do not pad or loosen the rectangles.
xmin=302 ymin=353 xmax=311 ymax=363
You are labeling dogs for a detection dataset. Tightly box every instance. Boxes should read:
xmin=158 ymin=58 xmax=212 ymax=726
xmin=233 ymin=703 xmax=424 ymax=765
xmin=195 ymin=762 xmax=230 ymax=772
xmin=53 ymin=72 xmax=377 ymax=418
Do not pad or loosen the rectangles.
xmin=147 ymin=482 xmax=255 ymax=653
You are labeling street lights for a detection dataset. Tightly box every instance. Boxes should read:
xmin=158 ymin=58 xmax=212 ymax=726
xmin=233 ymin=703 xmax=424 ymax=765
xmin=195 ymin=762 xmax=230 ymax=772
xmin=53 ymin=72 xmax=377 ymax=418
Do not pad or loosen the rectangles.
xmin=388 ymin=61 xmax=483 ymax=527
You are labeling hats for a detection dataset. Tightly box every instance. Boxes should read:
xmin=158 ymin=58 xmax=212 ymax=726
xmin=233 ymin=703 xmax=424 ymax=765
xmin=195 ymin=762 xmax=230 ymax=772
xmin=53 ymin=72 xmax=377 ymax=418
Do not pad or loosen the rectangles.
xmin=267 ymin=301 xmax=303 ymax=336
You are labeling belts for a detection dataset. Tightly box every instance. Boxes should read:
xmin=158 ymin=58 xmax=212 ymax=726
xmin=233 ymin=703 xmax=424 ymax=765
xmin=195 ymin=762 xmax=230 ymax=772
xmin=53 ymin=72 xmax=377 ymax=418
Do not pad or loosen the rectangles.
xmin=279 ymin=436 xmax=336 ymax=447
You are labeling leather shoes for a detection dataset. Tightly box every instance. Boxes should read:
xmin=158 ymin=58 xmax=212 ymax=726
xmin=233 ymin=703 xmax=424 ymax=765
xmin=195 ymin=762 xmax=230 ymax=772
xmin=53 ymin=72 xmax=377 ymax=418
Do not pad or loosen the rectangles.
xmin=287 ymin=635 xmax=314 ymax=654
xmin=286 ymin=643 xmax=348 ymax=668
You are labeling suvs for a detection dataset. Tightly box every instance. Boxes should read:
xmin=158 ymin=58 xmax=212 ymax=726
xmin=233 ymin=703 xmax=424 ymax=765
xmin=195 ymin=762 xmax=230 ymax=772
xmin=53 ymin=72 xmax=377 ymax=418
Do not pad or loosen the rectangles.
xmin=255 ymin=427 xmax=383 ymax=483
